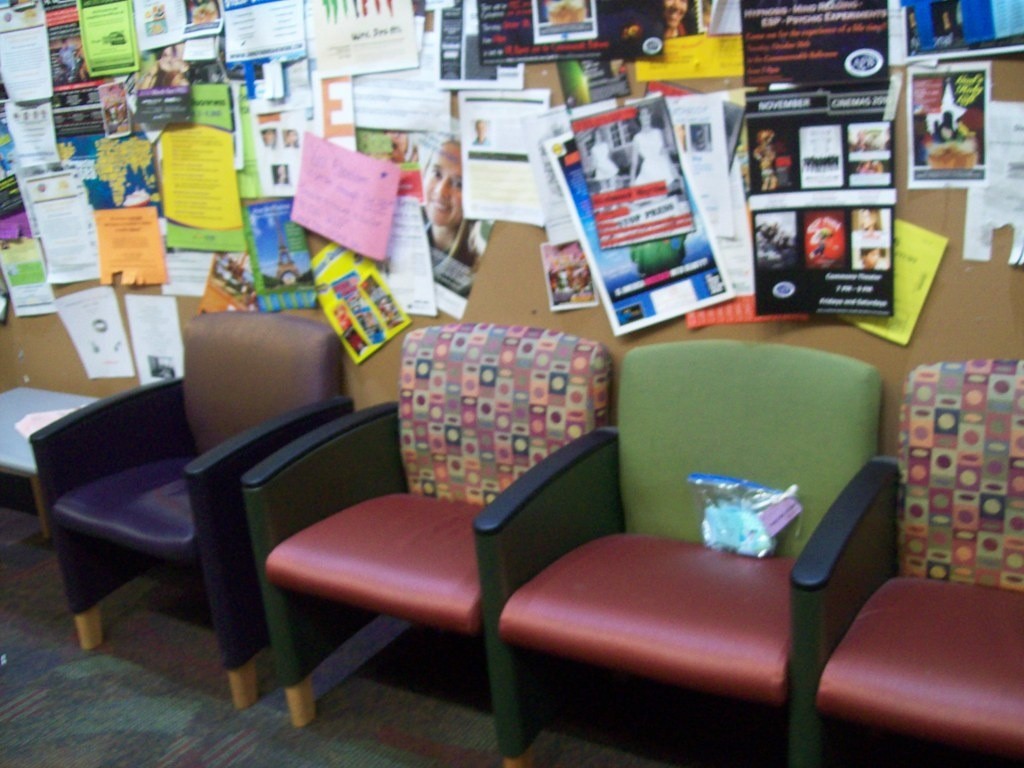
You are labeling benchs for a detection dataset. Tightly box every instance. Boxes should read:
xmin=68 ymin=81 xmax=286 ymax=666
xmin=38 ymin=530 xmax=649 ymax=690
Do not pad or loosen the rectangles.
xmin=239 ymin=311 xmax=1024 ymax=768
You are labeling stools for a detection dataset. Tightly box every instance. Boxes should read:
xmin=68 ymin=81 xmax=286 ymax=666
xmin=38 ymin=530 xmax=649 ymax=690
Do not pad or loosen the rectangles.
xmin=1 ymin=387 xmax=99 ymax=541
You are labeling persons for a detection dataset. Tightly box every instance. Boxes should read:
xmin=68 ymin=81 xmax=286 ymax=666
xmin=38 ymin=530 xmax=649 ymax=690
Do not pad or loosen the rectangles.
xmin=628 ymin=104 xmax=681 ymax=193
xmin=853 ymin=209 xmax=881 ymax=246
xmin=473 ymin=120 xmax=488 ymax=145
xmin=263 ymin=129 xmax=277 ymax=146
xmin=931 ymin=0 xmax=957 ymax=48
xmin=56 ymin=38 xmax=87 ymax=84
xmin=420 ymin=141 xmax=487 ymax=298
xmin=859 ymin=247 xmax=886 ymax=269
xmin=591 ymin=128 xmax=620 ymax=189
xmin=385 ymin=133 xmax=418 ymax=163
xmin=754 ymin=143 xmax=777 ymax=190
xmin=145 ymin=46 xmax=191 ymax=91
xmin=285 ymin=130 xmax=299 ymax=147
xmin=622 ymin=0 xmax=688 ymax=41
xmin=275 ymin=165 xmax=288 ymax=184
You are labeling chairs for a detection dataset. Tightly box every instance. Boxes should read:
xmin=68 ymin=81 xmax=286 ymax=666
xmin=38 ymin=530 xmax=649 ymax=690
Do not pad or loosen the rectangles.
xmin=22 ymin=311 xmax=351 ymax=711
